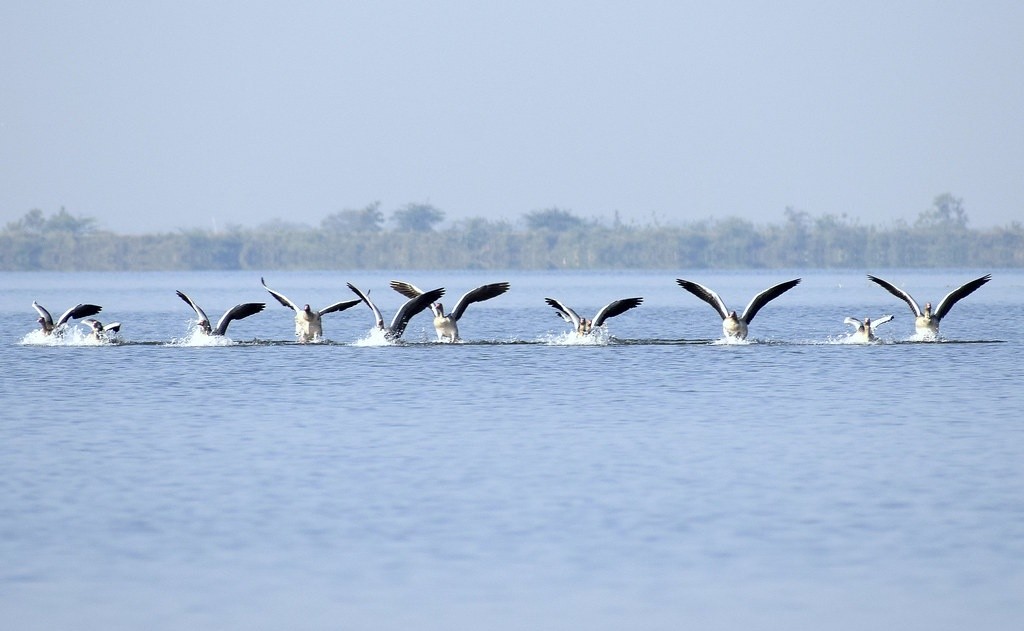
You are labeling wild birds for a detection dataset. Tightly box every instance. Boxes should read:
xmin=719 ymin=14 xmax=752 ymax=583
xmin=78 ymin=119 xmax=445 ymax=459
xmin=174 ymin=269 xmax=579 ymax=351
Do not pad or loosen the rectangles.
xmin=259 ymin=276 xmax=369 ymax=343
xmin=394 ymin=279 xmax=511 ymax=347
xmin=31 ymin=300 xmax=121 ymax=343
xmin=864 ymin=272 xmax=996 ymax=345
xmin=843 ymin=311 xmax=894 ymax=342
xmin=676 ymin=275 xmax=802 ymax=340
xmin=544 ymin=295 xmax=644 ymax=335
xmin=346 ymin=281 xmax=448 ymax=342
xmin=174 ymin=288 xmax=266 ymax=336
